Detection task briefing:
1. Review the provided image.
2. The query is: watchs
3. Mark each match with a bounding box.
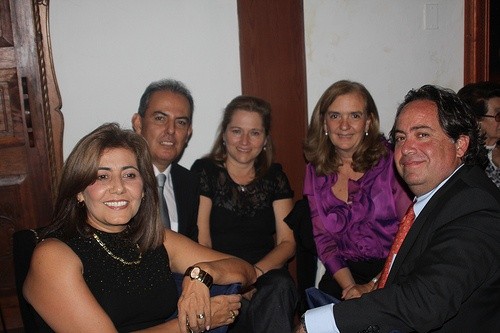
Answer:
[185,266,213,289]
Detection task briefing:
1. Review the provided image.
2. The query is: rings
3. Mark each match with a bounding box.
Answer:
[230,310,236,319]
[197,314,205,319]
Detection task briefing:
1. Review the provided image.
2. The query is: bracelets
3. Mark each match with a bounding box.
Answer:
[254,263,264,275]
[299,314,308,333]
[372,277,379,283]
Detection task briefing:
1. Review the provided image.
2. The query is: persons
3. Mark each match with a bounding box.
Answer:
[457,81,500,190]
[132,79,201,243]
[294,85,500,333]
[303,80,417,301]
[190,96,297,333]
[23,122,257,333]
[226,163,255,192]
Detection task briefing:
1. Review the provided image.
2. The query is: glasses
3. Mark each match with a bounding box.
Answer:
[482,113,500,122]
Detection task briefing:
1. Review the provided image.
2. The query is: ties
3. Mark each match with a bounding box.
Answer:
[375,199,418,289]
[155,174,171,228]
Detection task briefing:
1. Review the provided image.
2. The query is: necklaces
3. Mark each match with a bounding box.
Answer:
[92,224,142,265]
